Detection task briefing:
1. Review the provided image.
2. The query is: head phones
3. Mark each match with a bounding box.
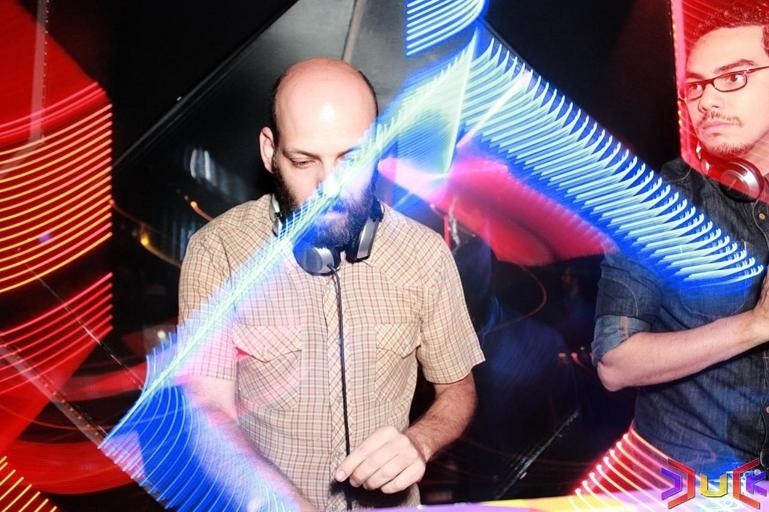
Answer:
[270,191,383,276]
[695,141,764,202]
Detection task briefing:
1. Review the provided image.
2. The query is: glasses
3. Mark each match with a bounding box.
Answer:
[682,66,769,100]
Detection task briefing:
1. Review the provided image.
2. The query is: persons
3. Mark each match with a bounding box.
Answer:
[170,52,489,512]
[579,0,769,485]
